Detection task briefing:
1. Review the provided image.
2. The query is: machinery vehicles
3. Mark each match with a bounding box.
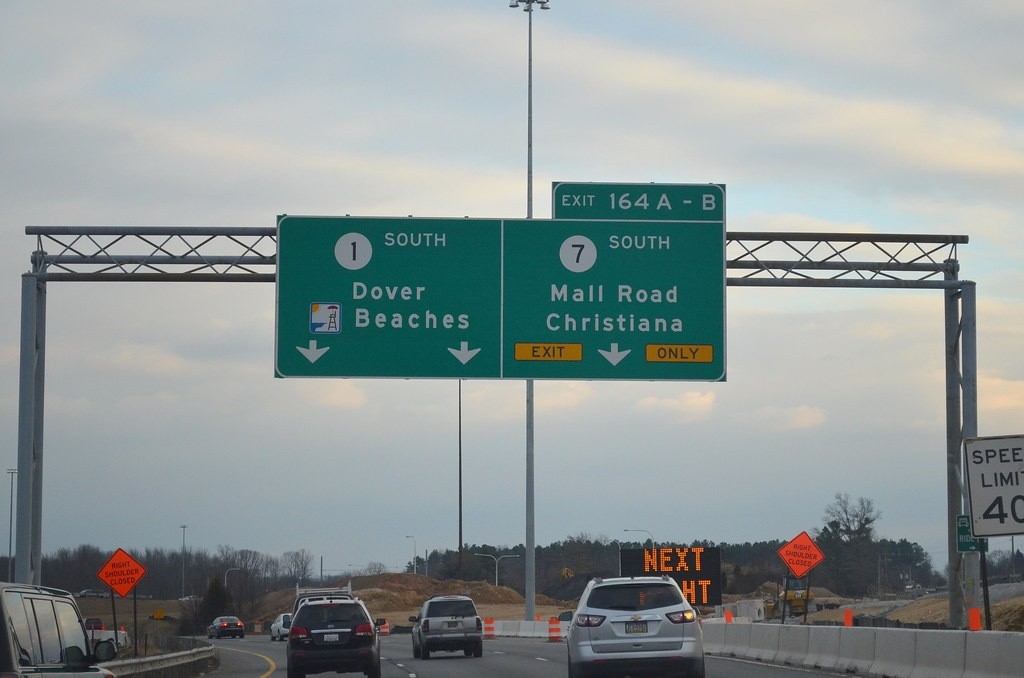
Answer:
[761,573,816,621]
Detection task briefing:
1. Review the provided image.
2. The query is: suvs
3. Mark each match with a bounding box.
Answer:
[408,593,484,660]
[558,572,705,678]
[282,590,386,678]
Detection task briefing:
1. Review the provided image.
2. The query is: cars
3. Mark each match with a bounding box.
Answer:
[74,587,154,602]
[207,616,245,640]
[270,612,294,641]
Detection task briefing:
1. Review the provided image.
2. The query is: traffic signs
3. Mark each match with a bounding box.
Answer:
[274,214,503,381]
[501,216,727,382]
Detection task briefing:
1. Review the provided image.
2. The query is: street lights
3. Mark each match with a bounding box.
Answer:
[877,557,892,597]
[224,567,241,588]
[623,529,655,549]
[6,469,19,581]
[180,524,188,598]
[406,534,417,574]
[347,564,367,577]
[474,553,520,587]
[605,539,622,577]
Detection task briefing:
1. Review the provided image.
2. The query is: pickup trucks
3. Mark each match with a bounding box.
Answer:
[0,581,132,678]
